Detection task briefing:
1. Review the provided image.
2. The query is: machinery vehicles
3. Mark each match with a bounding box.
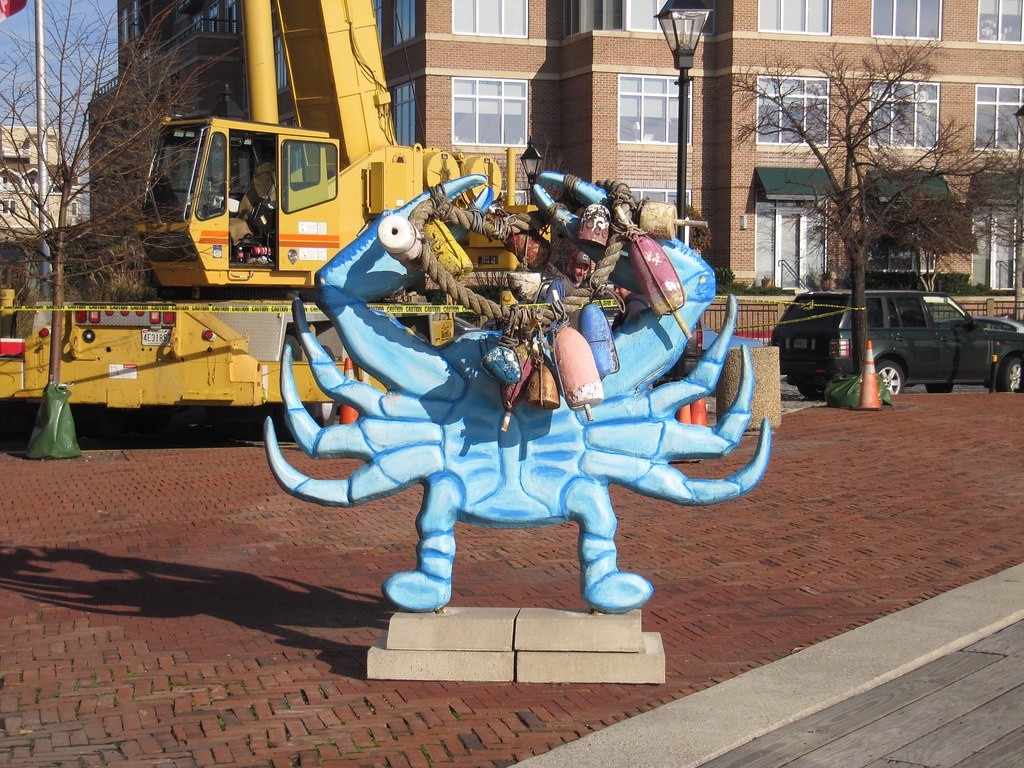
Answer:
[0,1,619,435]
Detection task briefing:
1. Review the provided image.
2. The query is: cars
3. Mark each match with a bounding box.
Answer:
[935,315,1023,332]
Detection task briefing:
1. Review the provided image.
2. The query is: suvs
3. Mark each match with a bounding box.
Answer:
[771,290,1024,394]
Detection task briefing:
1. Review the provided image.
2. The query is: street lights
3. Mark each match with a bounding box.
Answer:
[653,0,714,243]
[519,136,544,205]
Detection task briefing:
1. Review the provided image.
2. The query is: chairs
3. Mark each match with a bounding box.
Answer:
[228,158,277,247]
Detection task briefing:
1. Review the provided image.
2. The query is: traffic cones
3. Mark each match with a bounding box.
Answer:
[855,341,882,412]
